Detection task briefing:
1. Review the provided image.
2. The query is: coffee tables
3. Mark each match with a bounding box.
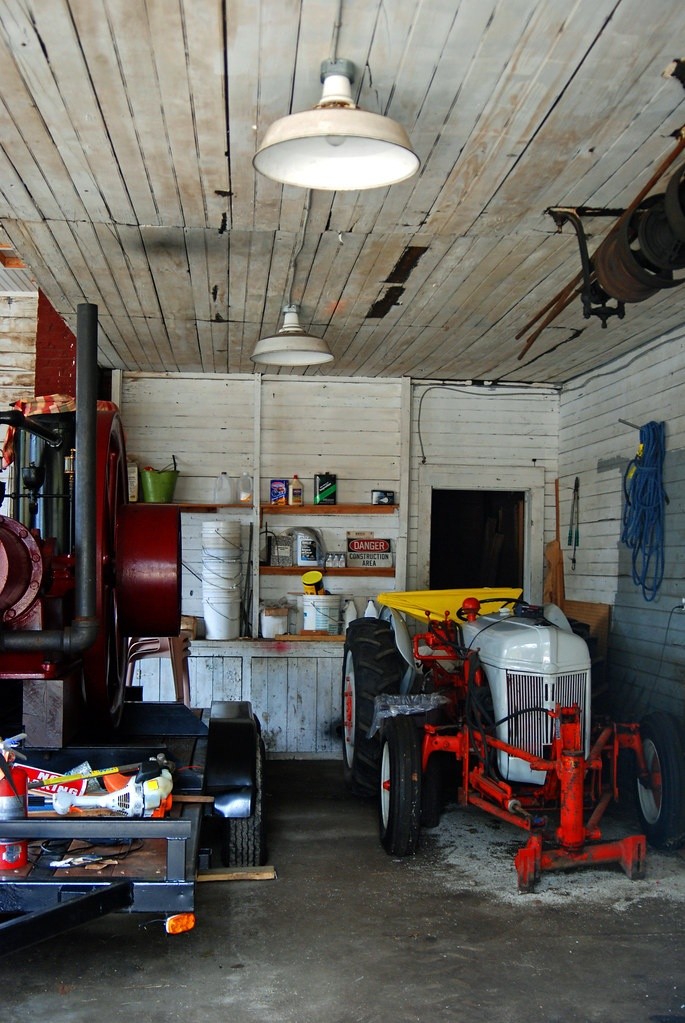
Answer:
[1,778,200,911]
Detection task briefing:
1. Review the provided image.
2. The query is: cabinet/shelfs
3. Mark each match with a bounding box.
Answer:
[255,499,398,641]
[118,498,253,641]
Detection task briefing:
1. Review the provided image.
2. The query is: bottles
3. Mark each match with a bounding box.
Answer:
[288,475,305,506]
[297,529,322,566]
[341,599,357,635]
[214,471,235,504]
[339,554,346,568]
[236,472,253,504]
[364,600,379,619]
[333,553,340,568]
[325,553,333,567]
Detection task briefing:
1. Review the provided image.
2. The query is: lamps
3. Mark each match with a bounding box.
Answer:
[250,304,334,367]
[253,57,421,191]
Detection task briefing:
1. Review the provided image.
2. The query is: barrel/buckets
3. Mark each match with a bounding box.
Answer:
[140,463,180,503]
[303,595,341,635]
[200,520,244,640]
